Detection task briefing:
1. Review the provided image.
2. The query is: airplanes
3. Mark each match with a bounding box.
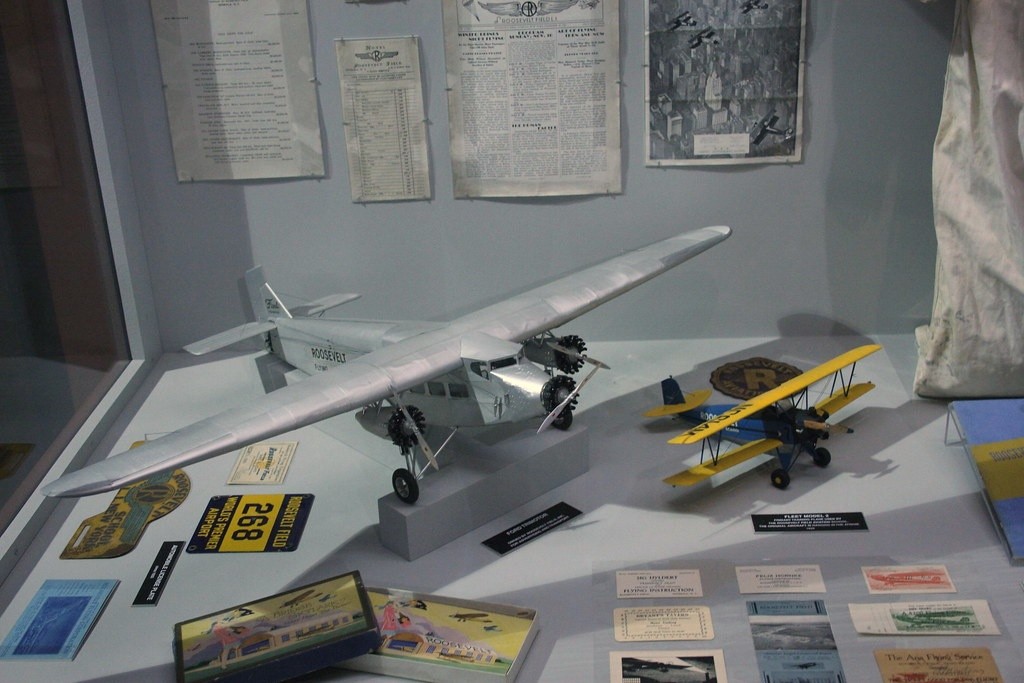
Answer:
[39,210,733,505]
[646,341,883,490]
